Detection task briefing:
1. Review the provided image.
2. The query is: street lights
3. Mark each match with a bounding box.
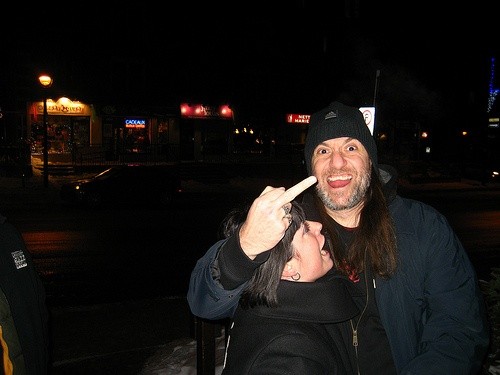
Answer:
[0,107,10,171]
[38,74,53,187]
[415,127,428,160]
[234,127,254,153]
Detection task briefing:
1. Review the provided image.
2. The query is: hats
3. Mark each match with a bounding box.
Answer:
[304,101,379,176]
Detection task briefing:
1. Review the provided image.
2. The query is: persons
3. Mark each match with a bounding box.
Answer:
[185,101,481,375]
[223,186,361,375]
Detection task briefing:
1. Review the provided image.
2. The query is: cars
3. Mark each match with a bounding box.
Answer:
[74,164,185,209]
